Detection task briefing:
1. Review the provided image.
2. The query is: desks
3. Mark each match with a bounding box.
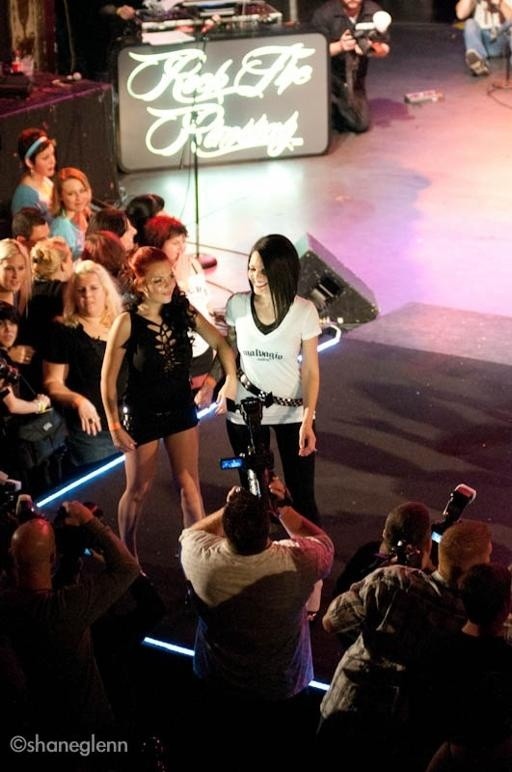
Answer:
[0,71,122,241]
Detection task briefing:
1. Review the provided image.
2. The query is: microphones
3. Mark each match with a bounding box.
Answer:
[491,17,511,45]
[49,72,82,86]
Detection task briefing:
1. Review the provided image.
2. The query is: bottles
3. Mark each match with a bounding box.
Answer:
[11,47,34,78]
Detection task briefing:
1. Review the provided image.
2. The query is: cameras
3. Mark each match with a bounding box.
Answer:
[199,14,222,40]
[55,498,101,547]
[430,483,476,562]
[348,19,388,58]
[0,356,20,385]
[219,391,274,498]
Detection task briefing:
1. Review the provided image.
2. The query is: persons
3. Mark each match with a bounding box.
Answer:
[1,0,512,769]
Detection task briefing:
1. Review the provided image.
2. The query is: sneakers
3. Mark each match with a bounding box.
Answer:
[465,49,489,75]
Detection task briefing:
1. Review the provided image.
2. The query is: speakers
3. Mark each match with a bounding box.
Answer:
[293,231,378,336]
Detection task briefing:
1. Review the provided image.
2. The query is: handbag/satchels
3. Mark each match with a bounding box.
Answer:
[20,411,69,463]
